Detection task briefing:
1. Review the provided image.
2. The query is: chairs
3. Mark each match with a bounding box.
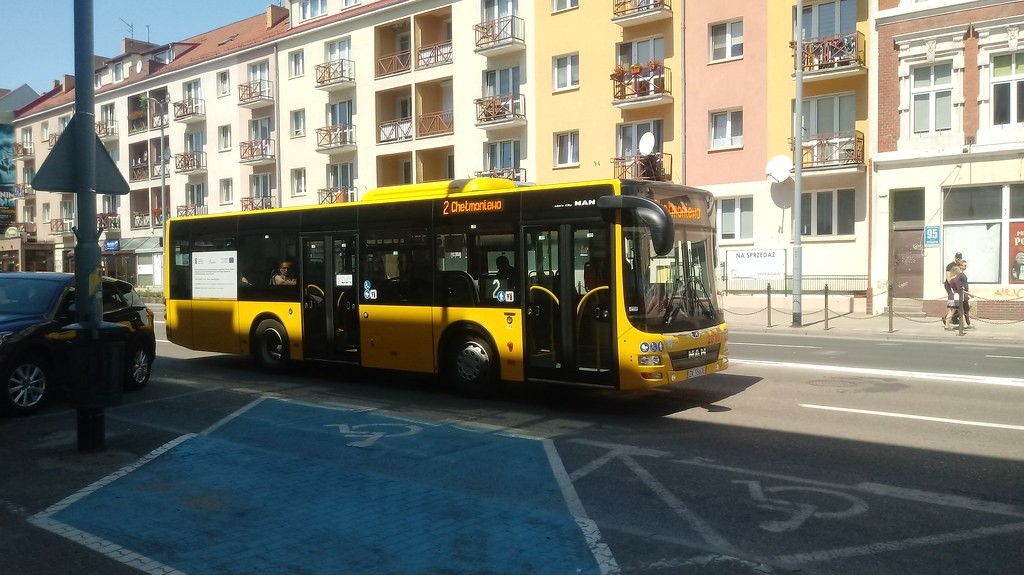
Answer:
[269,269,277,285]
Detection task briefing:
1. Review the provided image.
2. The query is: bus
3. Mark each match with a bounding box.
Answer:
[164,177,730,402]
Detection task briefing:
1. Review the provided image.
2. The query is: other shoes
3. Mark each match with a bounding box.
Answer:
[942,314,974,330]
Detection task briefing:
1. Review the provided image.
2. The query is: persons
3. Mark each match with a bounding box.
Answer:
[496,256,516,290]
[942,252,976,330]
[241,259,297,286]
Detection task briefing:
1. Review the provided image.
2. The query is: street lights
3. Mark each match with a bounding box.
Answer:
[141,96,167,250]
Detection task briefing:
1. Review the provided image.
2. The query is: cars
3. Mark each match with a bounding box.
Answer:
[0,272,157,418]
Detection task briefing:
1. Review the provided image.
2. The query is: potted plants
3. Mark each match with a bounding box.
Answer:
[647,59,658,71]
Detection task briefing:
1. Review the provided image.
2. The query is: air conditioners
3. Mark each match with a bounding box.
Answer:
[631,63,643,74]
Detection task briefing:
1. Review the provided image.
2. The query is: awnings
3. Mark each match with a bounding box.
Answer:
[69,237,163,256]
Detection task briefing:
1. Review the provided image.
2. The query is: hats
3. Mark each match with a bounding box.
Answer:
[955,252,962,258]
